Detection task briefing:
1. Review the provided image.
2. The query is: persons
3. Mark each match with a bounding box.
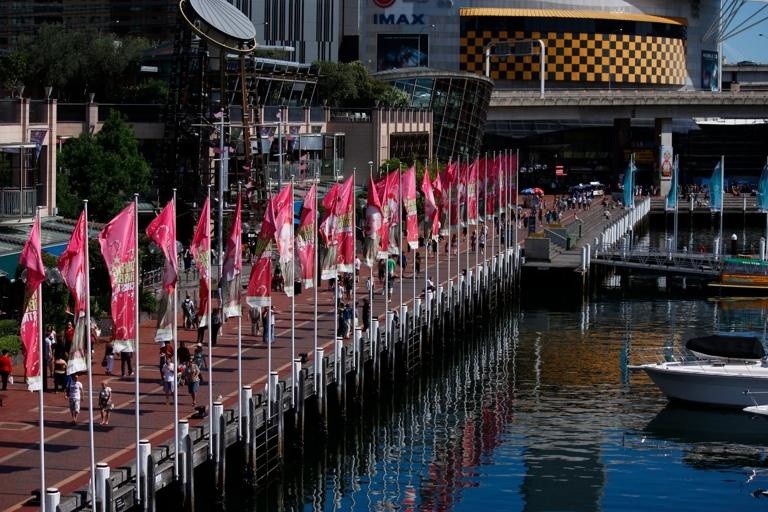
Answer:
[413,251,420,278]
[1,348,14,391]
[159,339,208,406]
[249,306,279,344]
[462,222,488,255]
[494,208,516,248]
[424,276,436,292]
[329,255,361,307]
[102,337,134,377]
[602,183,757,220]
[182,295,223,344]
[378,251,407,303]
[520,190,590,228]
[418,234,459,258]
[367,276,375,300]
[98,382,112,424]
[90,318,97,353]
[43,321,87,424]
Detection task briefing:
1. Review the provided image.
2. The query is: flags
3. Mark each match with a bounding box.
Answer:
[220,192,242,318]
[374,168,401,260]
[338,173,355,272]
[364,178,383,269]
[296,180,316,289]
[622,153,768,215]
[191,199,208,327]
[320,182,339,280]
[97,202,136,353]
[245,192,277,308]
[21,217,42,390]
[272,182,294,297]
[145,198,178,341]
[421,152,518,246]
[57,209,88,376]
[400,165,417,248]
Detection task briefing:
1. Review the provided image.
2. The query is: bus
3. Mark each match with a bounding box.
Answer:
[588,181,605,196]
[568,183,591,200]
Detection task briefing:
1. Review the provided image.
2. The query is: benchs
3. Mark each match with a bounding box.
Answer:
[560,199,622,239]
[694,192,760,208]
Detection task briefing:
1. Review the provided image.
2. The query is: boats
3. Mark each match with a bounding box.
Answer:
[623,401,767,471]
[706,257,768,289]
[740,391,767,418]
[625,331,767,407]
[706,296,767,329]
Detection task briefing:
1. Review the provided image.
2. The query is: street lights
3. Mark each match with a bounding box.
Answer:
[758,33,767,39]
[240,223,261,256]
[317,210,320,287]
[20,267,62,391]
[149,240,183,255]
[416,190,420,200]
[607,28,623,89]
[416,23,436,67]
